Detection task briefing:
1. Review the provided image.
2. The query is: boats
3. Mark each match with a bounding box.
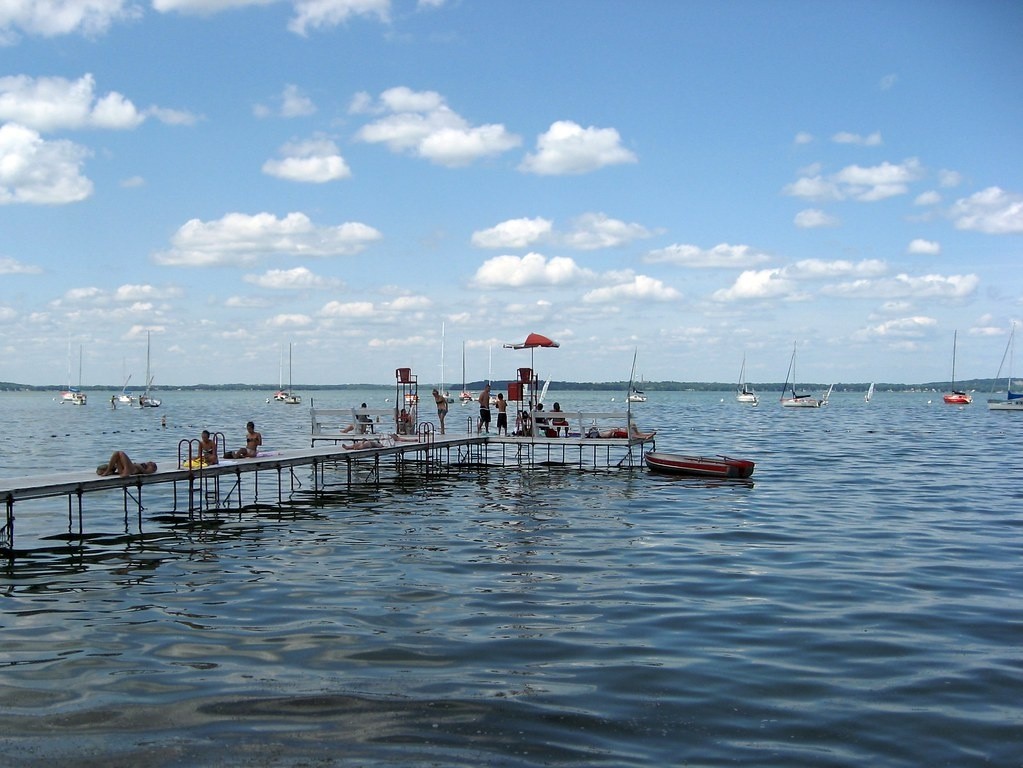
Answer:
[643,452,756,480]
[112,374,137,403]
[628,394,648,403]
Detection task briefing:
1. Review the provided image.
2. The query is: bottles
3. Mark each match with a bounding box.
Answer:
[376,415,379,422]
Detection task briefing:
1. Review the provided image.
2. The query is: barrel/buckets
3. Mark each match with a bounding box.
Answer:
[399,367,410,382]
[519,368,530,381]
[508,382,523,401]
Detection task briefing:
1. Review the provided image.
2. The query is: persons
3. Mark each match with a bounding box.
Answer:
[112,395,118,409]
[138,395,143,406]
[398,408,415,435]
[191,429,218,466]
[495,393,510,437]
[535,403,549,431]
[161,414,166,430]
[600,424,645,437]
[102,451,157,480]
[355,403,376,434]
[479,384,491,433]
[432,388,448,433]
[342,438,383,450]
[233,420,262,458]
[547,402,570,437]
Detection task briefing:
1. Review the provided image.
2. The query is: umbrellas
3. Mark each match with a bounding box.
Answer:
[503,333,561,411]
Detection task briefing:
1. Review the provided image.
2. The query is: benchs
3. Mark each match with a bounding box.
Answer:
[308,408,400,436]
[531,408,635,440]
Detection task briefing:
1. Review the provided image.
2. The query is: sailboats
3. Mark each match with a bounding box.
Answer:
[274,342,301,405]
[139,330,162,407]
[438,321,455,403]
[404,374,420,403]
[61,332,87,405]
[985,321,1023,410]
[459,341,543,406]
[780,341,823,407]
[943,330,973,403]
[735,359,757,402]
